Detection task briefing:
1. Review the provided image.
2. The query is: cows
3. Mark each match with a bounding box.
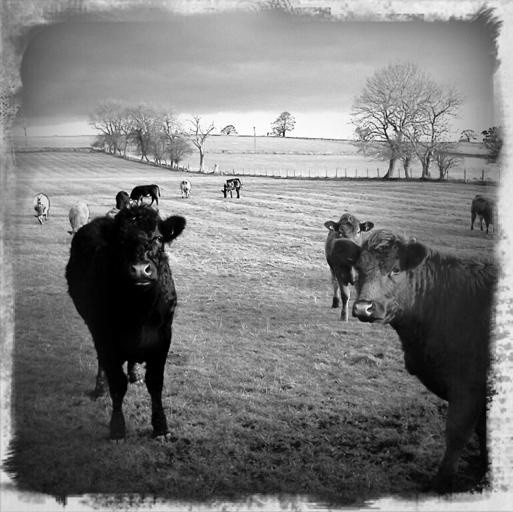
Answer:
[129,184,161,206]
[67,202,90,235]
[32,192,50,225]
[330,228,498,497]
[115,191,130,211]
[64,204,187,447]
[470,193,497,234]
[220,178,243,199]
[323,213,375,323]
[180,180,191,199]
